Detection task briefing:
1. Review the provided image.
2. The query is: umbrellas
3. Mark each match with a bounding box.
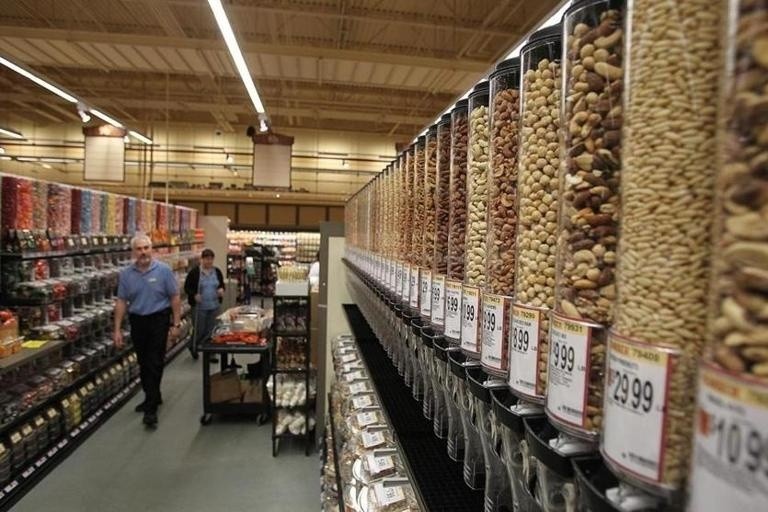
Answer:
[189,347,198,359]
[209,358,219,364]
[136,397,162,412]
[144,410,158,425]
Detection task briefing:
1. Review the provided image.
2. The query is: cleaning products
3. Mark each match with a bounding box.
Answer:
[196,318,272,427]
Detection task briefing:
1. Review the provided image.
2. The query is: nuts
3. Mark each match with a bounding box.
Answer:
[379,0,768,485]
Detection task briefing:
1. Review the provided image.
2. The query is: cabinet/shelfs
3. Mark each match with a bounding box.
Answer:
[221,229,321,283]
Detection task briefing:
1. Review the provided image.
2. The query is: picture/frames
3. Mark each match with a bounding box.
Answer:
[327,304,482,512]
[198,331,274,426]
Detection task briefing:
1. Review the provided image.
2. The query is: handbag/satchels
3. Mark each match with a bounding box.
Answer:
[174,323,181,328]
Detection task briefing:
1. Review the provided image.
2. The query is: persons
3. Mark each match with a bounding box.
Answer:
[183,248,227,363]
[111,233,183,428]
[309,247,320,297]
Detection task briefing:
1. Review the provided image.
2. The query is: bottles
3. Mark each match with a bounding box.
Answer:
[322,1,768,511]
[202,249,215,258]
[221,229,321,283]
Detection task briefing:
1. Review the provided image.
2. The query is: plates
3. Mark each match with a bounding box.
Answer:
[1,241,205,512]
[272,294,311,458]
